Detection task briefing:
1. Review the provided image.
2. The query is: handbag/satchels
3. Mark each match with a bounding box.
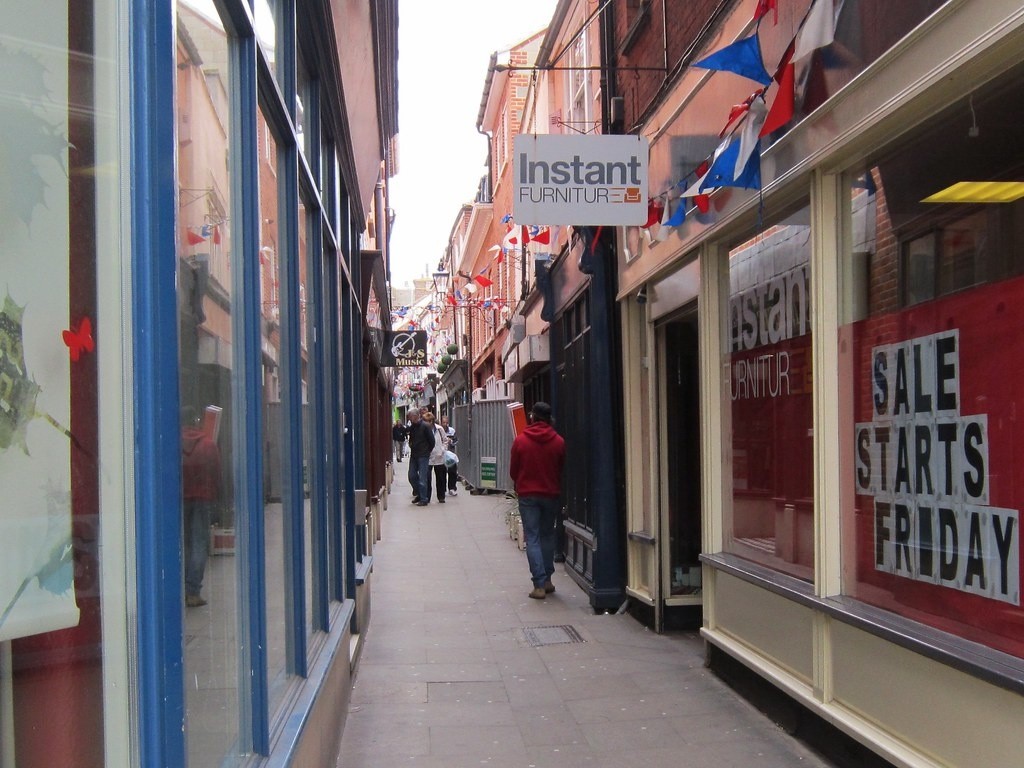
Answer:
[442,447,459,470]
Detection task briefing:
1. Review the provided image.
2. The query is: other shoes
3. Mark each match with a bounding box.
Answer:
[528,588,546,599]
[397,459,402,462]
[545,581,555,593]
[449,489,458,496]
[417,501,427,506]
[439,497,445,503]
[411,496,421,503]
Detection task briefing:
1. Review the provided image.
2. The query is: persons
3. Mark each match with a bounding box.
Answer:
[408,406,448,507]
[179,405,221,608]
[393,420,408,462]
[441,415,459,497]
[510,401,567,599]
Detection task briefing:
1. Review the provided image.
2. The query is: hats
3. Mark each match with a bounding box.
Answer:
[527,402,552,418]
